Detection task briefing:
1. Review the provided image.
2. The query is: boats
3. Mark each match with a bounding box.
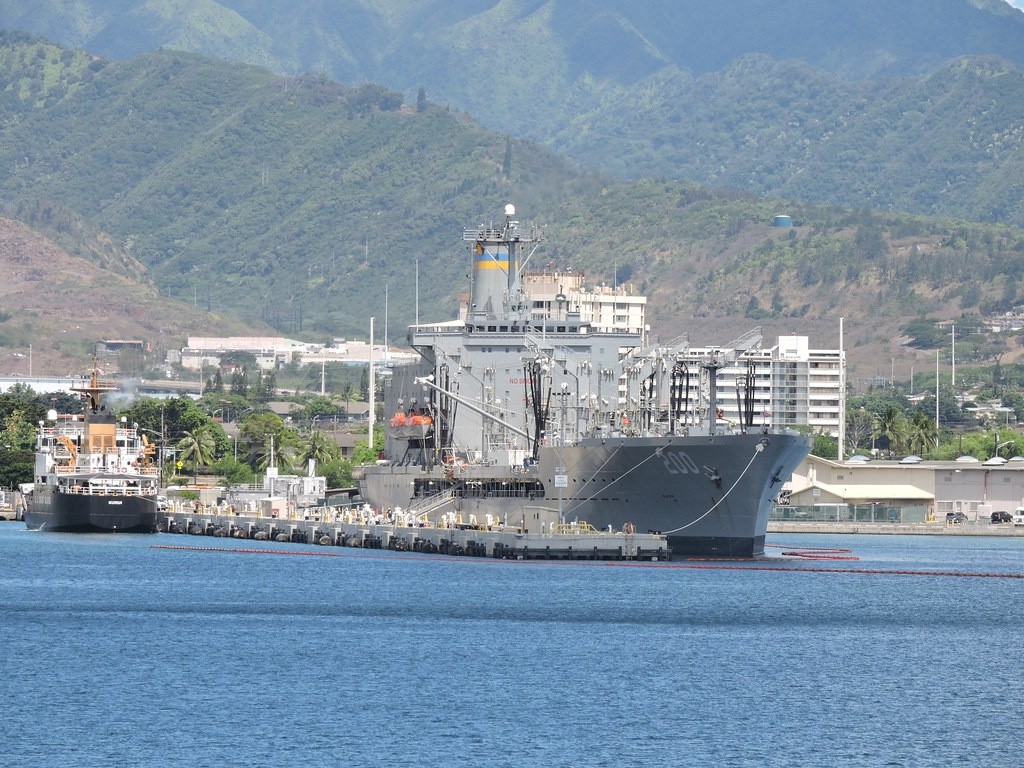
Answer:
[21,343,165,534]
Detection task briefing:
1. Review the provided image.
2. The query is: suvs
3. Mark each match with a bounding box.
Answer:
[991,511,1013,523]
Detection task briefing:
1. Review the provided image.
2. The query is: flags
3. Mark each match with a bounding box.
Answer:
[477,242,485,256]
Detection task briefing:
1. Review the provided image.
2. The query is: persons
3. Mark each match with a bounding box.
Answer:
[303,506,309,520]
[387,508,392,519]
[194,501,200,513]
[314,509,320,521]
[272,510,278,518]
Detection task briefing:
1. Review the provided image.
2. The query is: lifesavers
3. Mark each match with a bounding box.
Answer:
[121,467,127,473]
[272,510,278,517]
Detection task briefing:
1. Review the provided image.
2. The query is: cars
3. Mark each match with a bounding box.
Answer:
[945,512,968,523]
[1013,506,1024,527]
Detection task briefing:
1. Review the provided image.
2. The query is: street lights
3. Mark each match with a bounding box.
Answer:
[995,440,1015,457]
[205,403,340,467]
[141,427,164,489]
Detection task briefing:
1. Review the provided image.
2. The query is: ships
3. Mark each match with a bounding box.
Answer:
[357,199,816,559]
